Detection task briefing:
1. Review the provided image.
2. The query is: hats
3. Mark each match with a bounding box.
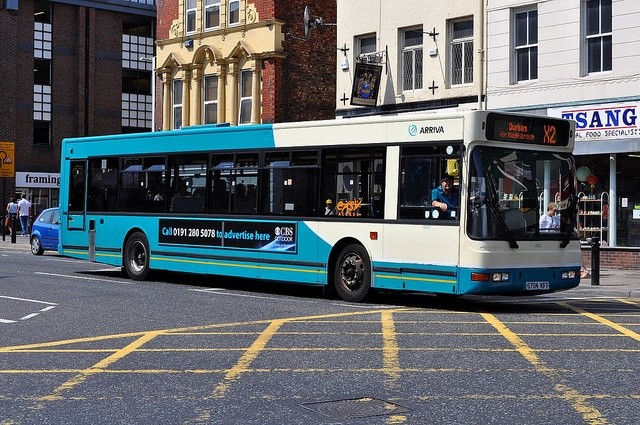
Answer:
[441,173,454,183]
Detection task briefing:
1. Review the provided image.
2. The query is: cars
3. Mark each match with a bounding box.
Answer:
[30,207,59,254]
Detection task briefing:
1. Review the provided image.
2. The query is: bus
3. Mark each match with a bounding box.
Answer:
[58,110,584,301]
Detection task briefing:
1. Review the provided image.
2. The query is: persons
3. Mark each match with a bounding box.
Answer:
[144,174,192,212]
[431,174,460,211]
[214,179,234,209]
[4,195,19,237]
[324,198,336,216]
[16,193,32,237]
[235,183,250,201]
[539,202,558,232]
